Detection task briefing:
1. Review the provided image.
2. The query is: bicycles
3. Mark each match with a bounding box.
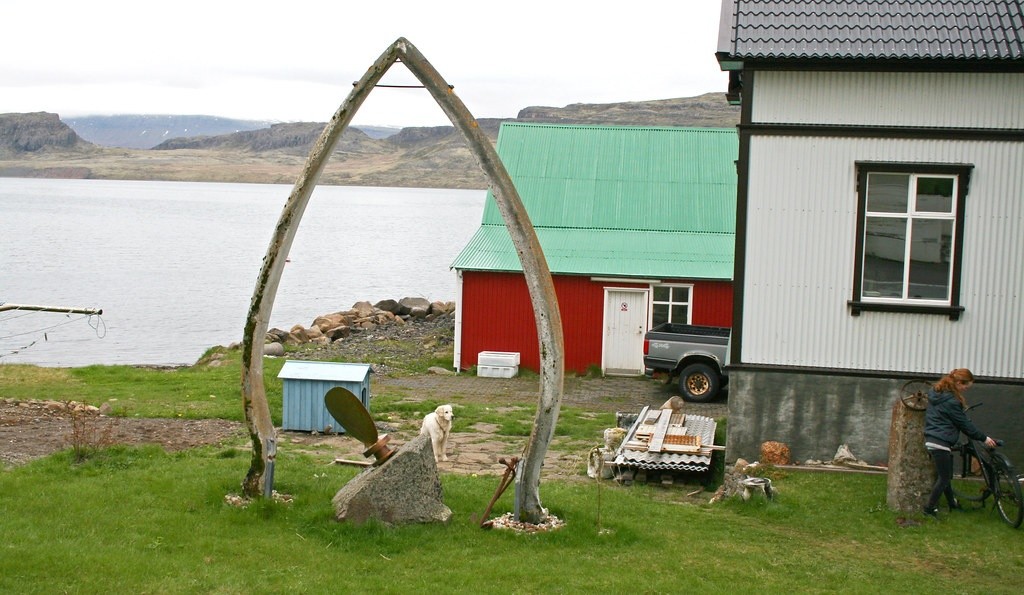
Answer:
[946,402,1024,532]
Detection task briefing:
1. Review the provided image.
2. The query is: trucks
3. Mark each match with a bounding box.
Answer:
[643,321,730,402]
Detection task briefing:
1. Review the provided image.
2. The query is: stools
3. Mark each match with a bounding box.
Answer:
[740,477,773,505]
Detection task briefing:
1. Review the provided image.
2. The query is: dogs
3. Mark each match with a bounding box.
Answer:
[420,404,454,463]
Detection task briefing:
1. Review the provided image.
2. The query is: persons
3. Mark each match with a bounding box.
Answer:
[922,369,996,522]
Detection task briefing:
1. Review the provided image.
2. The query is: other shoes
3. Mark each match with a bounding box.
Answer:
[920,506,941,519]
[949,499,965,512]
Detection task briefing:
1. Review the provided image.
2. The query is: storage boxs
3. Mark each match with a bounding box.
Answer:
[476,366,519,378]
[477,351,522,367]
